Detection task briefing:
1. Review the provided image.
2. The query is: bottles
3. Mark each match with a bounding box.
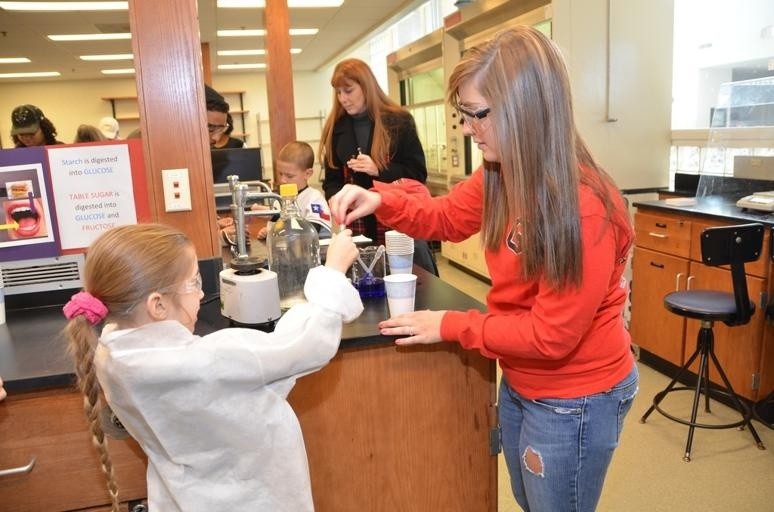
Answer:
[266,185,319,309]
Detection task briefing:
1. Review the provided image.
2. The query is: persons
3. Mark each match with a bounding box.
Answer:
[254,141,330,242]
[72,124,109,145]
[65,220,366,511]
[203,83,230,146]
[8,104,65,148]
[316,57,440,278]
[329,24,641,512]
[212,113,251,149]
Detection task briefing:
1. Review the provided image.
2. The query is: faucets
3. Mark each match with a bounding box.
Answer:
[235,184,284,256]
[227,174,274,204]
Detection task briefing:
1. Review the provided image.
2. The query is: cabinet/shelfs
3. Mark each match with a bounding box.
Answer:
[0,325,500,511]
[101,87,247,151]
[630,196,774,421]
[256,107,327,173]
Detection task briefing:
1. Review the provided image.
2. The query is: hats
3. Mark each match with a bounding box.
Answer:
[11,104,43,134]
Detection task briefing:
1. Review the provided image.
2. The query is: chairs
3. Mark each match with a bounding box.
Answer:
[637,221,766,460]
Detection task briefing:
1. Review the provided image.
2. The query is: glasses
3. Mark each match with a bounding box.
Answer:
[209,123,230,134]
[457,105,492,135]
[124,274,202,314]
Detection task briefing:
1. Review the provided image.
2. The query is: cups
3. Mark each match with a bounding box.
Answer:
[350,245,386,297]
[384,228,415,274]
[381,272,418,317]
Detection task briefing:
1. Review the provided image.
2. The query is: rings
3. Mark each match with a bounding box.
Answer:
[410,326,415,336]
